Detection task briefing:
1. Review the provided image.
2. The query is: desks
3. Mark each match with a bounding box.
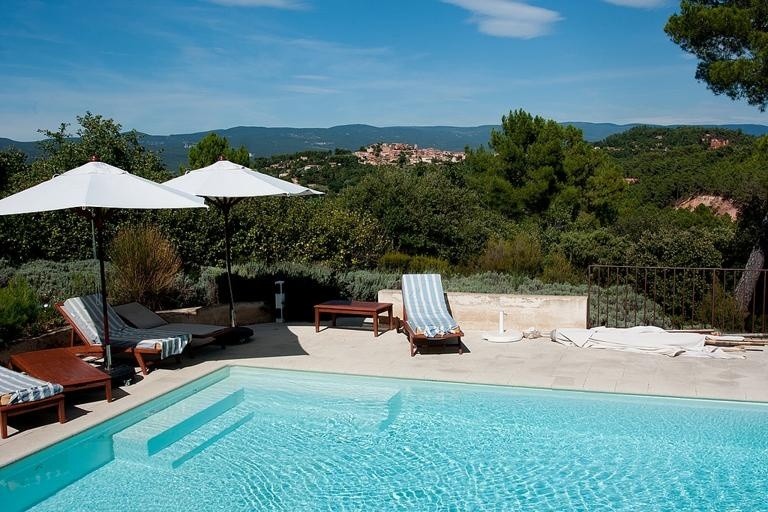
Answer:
[10,346,113,403]
[313,299,394,338]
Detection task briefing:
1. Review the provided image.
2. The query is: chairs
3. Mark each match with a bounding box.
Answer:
[398,272,464,356]
[0,363,68,440]
[54,291,255,376]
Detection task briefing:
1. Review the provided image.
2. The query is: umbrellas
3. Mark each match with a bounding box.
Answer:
[0,156,211,372]
[160,156,326,327]
